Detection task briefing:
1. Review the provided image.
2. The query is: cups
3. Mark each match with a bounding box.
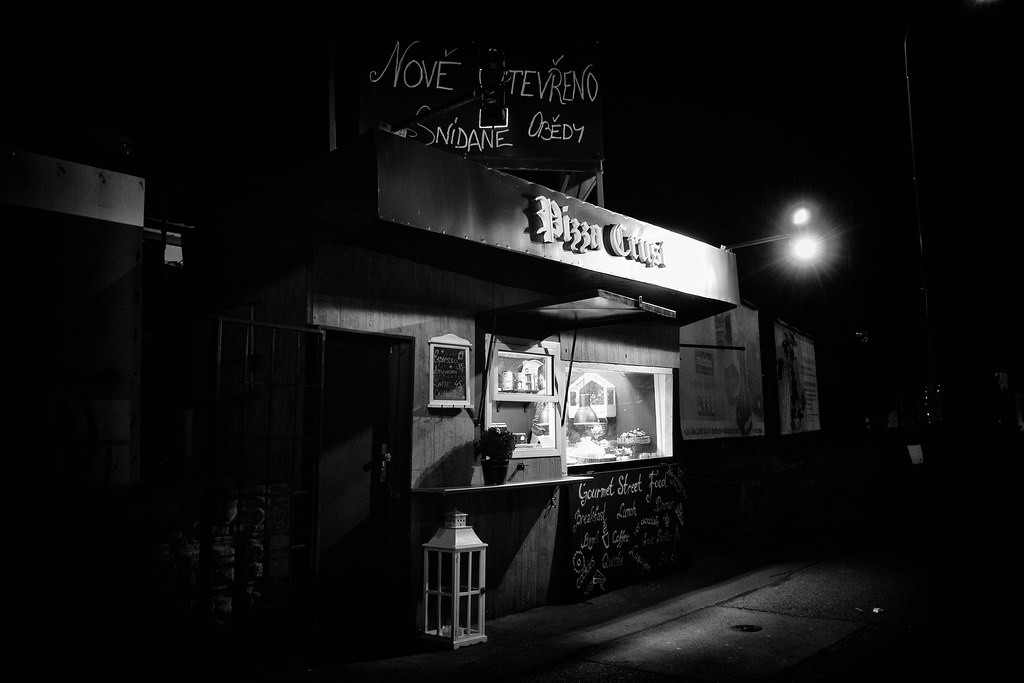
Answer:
[498,370,514,391]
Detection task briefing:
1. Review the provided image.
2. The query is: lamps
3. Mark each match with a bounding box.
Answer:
[573,373,598,424]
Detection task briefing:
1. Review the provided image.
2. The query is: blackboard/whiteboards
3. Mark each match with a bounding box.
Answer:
[365,1,607,173]
[564,463,686,605]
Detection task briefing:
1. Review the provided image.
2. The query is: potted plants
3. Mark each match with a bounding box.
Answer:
[474,428,517,485]
